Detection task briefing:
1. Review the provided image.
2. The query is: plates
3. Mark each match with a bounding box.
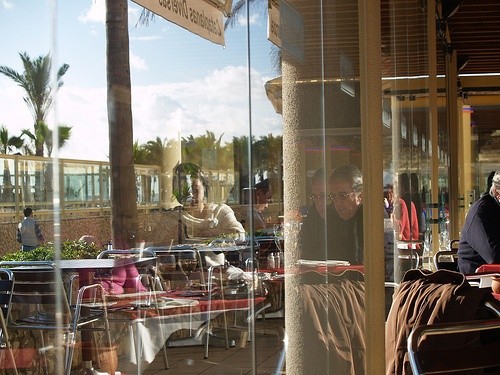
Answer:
[129,299,176,306]
[220,288,260,299]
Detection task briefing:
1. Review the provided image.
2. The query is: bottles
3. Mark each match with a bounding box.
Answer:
[269,252,275,269]
[274,252,280,269]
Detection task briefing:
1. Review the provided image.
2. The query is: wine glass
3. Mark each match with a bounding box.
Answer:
[156,254,176,299]
[179,251,198,301]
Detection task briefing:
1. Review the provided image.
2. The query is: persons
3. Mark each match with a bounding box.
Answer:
[457,171,500,277]
[439,186,448,221]
[154,162,275,325]
[302,166,422,278]
[16,208,46,252]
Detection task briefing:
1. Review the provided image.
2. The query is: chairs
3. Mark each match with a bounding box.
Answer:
[432,240,460,272]
[384,269,500,375]
[0,237,284,375]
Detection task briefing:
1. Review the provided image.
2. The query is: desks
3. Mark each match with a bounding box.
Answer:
[147,246,251,348]
[10,257,158,375]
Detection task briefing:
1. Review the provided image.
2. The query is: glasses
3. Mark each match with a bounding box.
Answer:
[311,194,327,200]
[328,192,352,200]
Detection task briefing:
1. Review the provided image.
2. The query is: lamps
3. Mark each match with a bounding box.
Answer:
[456,56,468,71]
[442,0,462,19]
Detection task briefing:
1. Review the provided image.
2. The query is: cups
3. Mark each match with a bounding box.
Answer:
[490,275,500,294]
[273,224,283,254]
